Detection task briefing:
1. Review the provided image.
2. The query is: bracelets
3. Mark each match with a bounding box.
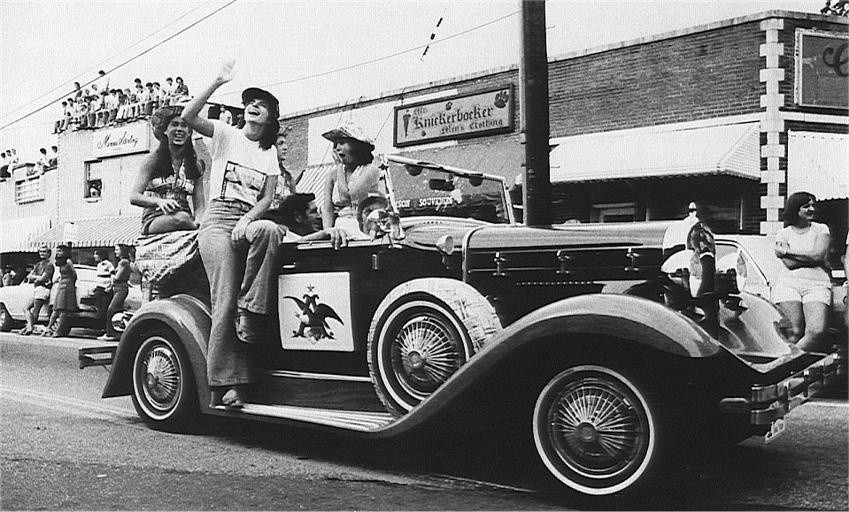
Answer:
[244,213,253,222]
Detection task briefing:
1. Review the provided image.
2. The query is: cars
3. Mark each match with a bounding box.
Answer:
[75,154,840,510]
[0,262,150,333]
[712,231,849,397]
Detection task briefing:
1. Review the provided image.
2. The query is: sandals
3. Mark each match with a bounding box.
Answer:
[222,387,243,408]
[233,308,256,344]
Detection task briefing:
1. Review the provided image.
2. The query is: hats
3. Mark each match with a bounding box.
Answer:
[242,88,279,106]
[151,105,185,141]
[358,191,388,212]
[321,122,376,151]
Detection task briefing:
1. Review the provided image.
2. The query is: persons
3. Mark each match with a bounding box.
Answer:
[0,244,131,341]
[403,109,411,136]
[774,192,831,352]
[281,193,348,251]
[129,106,206,234]
[322,125,390,242]
[0,71,245,183]
[259,126,296,226]
[181,58,285,409]
